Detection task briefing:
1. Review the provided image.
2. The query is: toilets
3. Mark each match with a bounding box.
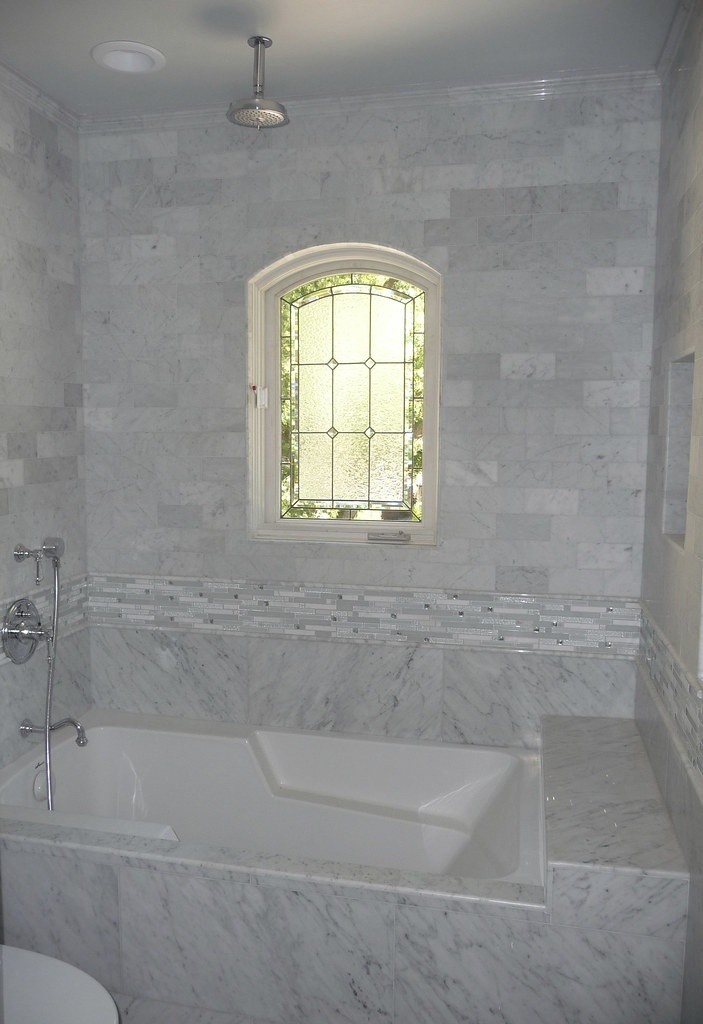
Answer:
[0,945,120,1024]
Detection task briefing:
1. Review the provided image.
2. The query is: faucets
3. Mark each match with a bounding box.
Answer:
[19,715,88,747]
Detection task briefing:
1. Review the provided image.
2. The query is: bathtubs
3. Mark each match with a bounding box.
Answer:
[0,709,544,1024]
